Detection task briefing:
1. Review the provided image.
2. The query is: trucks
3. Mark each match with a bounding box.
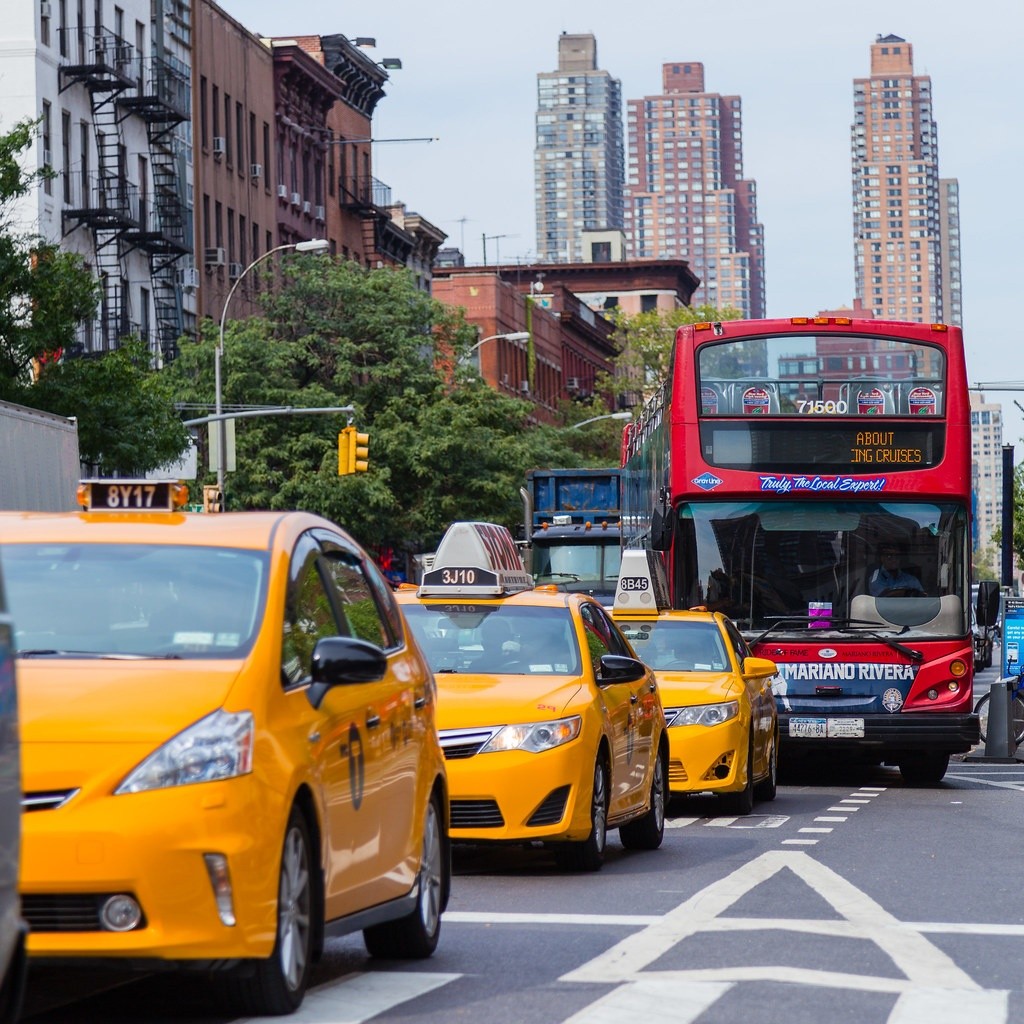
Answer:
[518,466,661,620]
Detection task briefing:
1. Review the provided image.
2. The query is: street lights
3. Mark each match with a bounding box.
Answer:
[448,332,531,393]
[217,238,331,499]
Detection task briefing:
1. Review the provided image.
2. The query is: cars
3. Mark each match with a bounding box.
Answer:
[971,583,1014,672]
[0,477,454,1016]
[590,549,780,819]
[378,520,673,874]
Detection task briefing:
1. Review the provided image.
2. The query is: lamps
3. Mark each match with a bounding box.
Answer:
[349,37,375,49]
[376,58,401,69]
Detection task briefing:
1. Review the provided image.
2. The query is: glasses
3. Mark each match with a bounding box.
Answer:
[883,553,901,560]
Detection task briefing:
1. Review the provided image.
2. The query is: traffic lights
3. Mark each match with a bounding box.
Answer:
[348,428,371,474]
[202,484,223,514]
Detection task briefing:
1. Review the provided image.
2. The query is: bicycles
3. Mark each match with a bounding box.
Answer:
[973,655,1024,745]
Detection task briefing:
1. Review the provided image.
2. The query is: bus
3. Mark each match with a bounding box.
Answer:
[618,313,1001,789]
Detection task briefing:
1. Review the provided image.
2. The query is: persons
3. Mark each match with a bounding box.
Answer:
[468,619,530,673]
[851,538,927,600]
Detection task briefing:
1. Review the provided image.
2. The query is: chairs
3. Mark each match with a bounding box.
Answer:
[840,377,944,416]
[626,376,782,465]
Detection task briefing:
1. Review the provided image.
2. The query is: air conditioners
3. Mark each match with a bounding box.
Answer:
[521,381,528,391]
[94,36,106,50]
[186,268,200,288]
[567,377,579,387]
[303,201,312,214]
[251,164,261,177]
[205,248,226,266]
[115,48,132,60]
[278,185,287,198]
[214,137,226,153]
[292,192,300,205]
[229,263,243,278]
[315,206,325,220]
